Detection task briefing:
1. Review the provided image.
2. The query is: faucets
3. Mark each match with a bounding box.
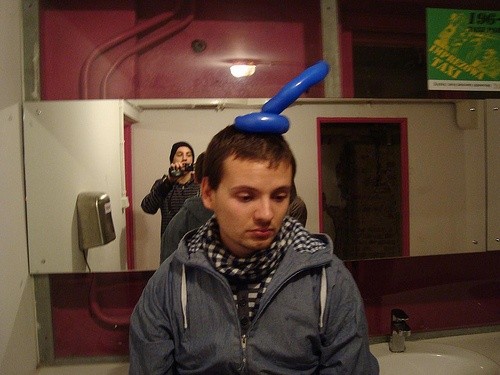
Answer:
[388,308,412,353]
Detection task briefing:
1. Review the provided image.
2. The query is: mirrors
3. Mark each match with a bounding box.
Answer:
[20,0,500,277]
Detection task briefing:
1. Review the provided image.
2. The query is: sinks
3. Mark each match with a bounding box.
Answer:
[368,342,499,375]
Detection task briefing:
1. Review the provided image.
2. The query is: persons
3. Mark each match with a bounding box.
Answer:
[129,123,380,375]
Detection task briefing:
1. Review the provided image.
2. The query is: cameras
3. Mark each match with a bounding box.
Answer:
[184,163,195,171]
